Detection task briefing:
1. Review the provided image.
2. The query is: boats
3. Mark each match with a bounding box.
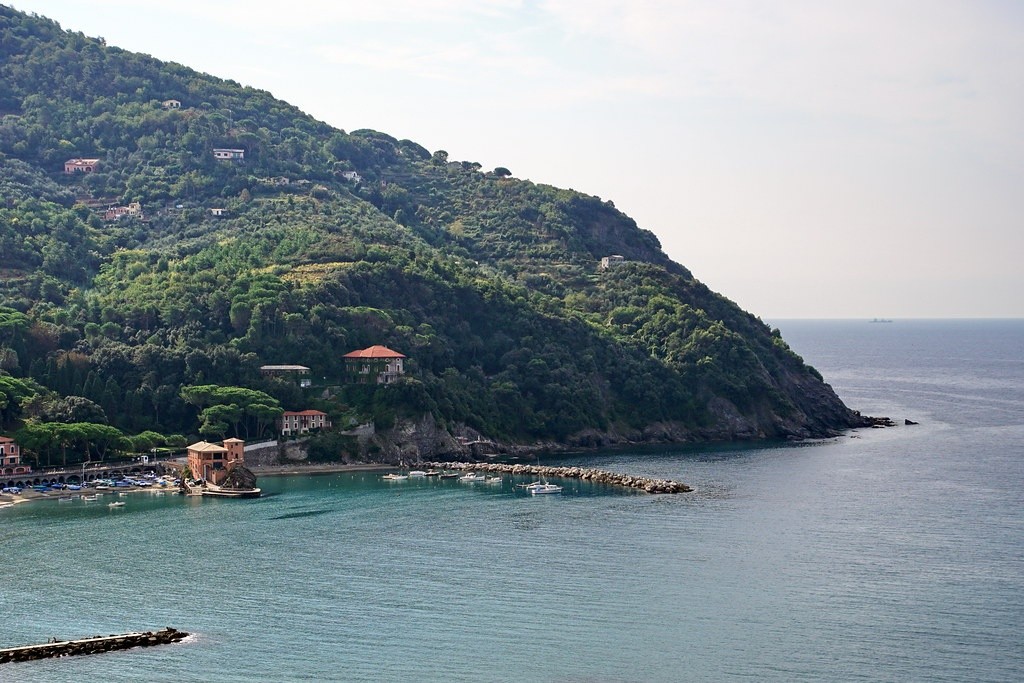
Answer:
[438,473,458,478]
[59,496,72,501]
[82,496,97,500]
[459,472,502,483]
[408,469,439,476]
[107,501,126,507]
[381,473,408,479]
[516,481,564,493]
